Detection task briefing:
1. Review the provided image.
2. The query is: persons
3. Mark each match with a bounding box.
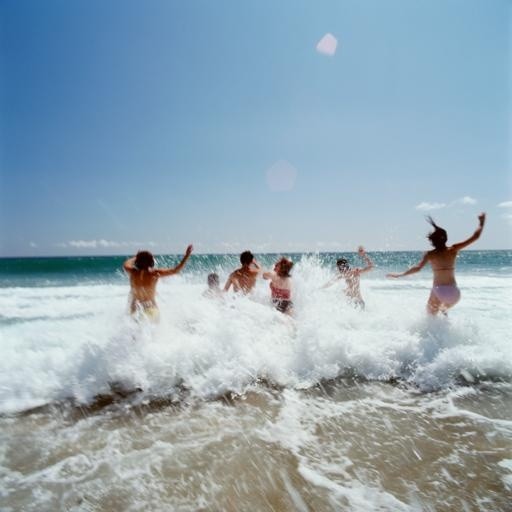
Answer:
[326,245,374,310]
[202,273,224,298]
[264,258,295,315]
[224,250,261,296]
[125,244,194,318]
[387,214,485,317]
